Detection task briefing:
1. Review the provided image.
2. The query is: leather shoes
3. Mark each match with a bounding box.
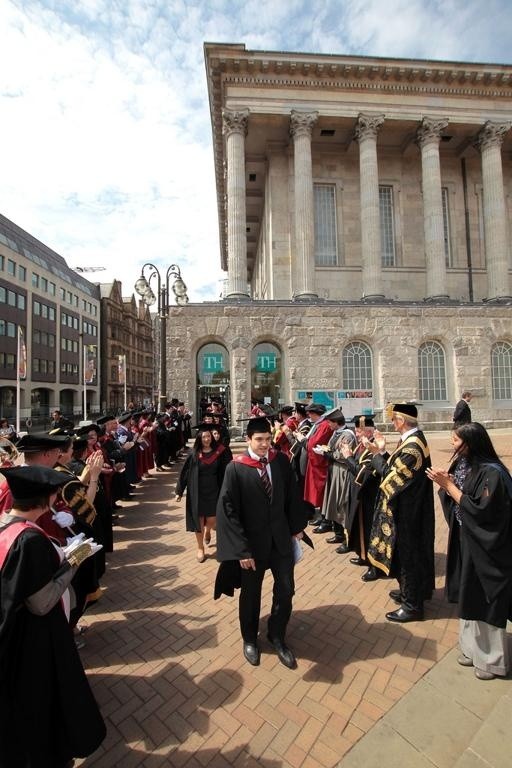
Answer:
[385,605,419,622]
[243,640,261,667]
[312,521,332,533]
[269,635,296,669]
[361,566,381,581]
[308,518,321,526]
[476,667,494,680]
[456,655,473,669]
[390,590,402,602]
[349,557,368,565]
[326,535,343,544]
[335,545,353,554]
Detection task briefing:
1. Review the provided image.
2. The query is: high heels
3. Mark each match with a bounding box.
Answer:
[205,535,210,545]
[195,549,207,562]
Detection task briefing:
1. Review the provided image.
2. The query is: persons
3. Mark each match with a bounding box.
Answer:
[83,424,115,553]
[51,410,73,432]
[129,401,194,486]
[98,415,124,512]
[118,416,136,502]
[107,415,132,501]
[196,401,232,536]
[311,408,360,554]
[450,391,474,428]
[293,403,332,534]
[64,428,107,578]
[0,418,19,457]
[216,418,304,671]
[52,432,104,635]
[177,424,227,565]
[362,402,435,621]
[0,433,94,656]
[245,403,311,463]
[339,414,385,581]
[427,422,511,681]
[0,466,111,768]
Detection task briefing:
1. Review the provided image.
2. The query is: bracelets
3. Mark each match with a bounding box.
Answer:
[89,480,99,483]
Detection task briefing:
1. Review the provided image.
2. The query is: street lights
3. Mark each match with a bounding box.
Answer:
[134,263,189,412]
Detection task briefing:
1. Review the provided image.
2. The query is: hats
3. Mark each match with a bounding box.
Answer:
[15,432,70,453]
[190,423,214,430]
[85,424,101,433]
[294,401,308,412]
[236,414,275,433]
[117,413,132,423]
[278,406,294,415]
[172,399,178,405]
[133,410,157,418]
[393,401,417,420]
[96,415,115,426]
[314,405,344,425]
[164,401,172,407]
[305,403,325,413]
[212,422,223,429]
[206,412,224,417]
[0,465,74,508]
[351,414,376,427]
[179,401,184,407]
